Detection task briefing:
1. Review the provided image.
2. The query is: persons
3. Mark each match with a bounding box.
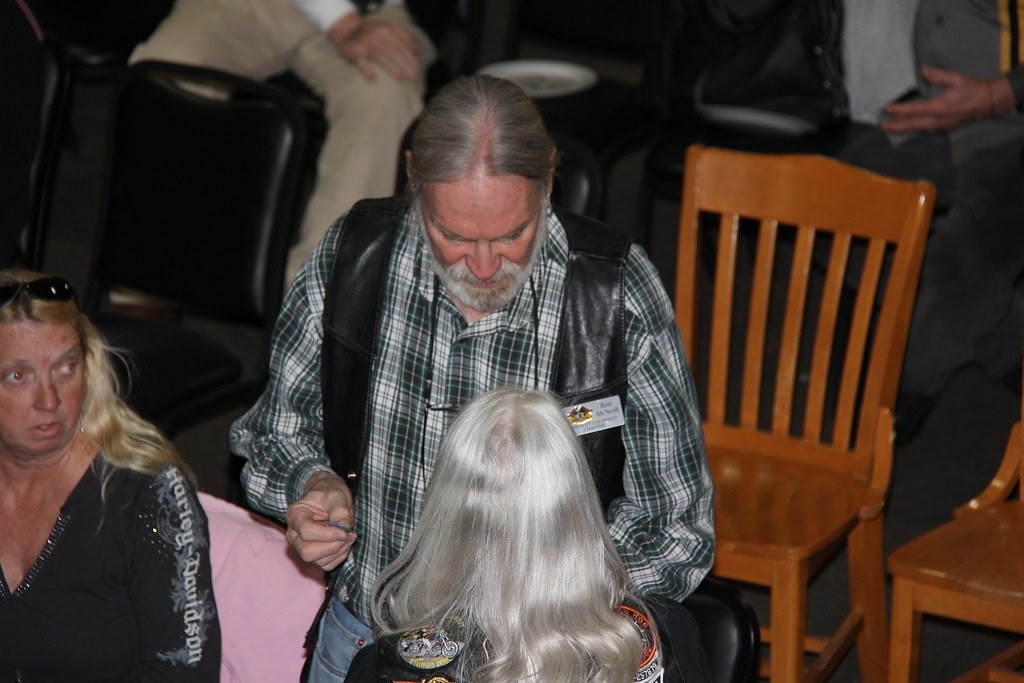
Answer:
[0,265,225,683]
[592,0,1024,610]
[230,72,717,683]
[124,0,438,300]
[340,387,716,683]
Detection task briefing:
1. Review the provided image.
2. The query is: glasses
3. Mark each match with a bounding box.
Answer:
[419,398,462,489]
[1,274,76,306]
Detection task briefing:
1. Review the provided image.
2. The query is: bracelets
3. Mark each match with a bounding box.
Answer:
[984,80,1000,119]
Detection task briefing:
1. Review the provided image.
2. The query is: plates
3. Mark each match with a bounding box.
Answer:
[476,58,599,100]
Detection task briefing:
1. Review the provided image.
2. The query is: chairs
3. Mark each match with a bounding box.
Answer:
[682,595,761,683]
[675,145,939,683]
[887,363,1024,683]
[78,58,328,443]
